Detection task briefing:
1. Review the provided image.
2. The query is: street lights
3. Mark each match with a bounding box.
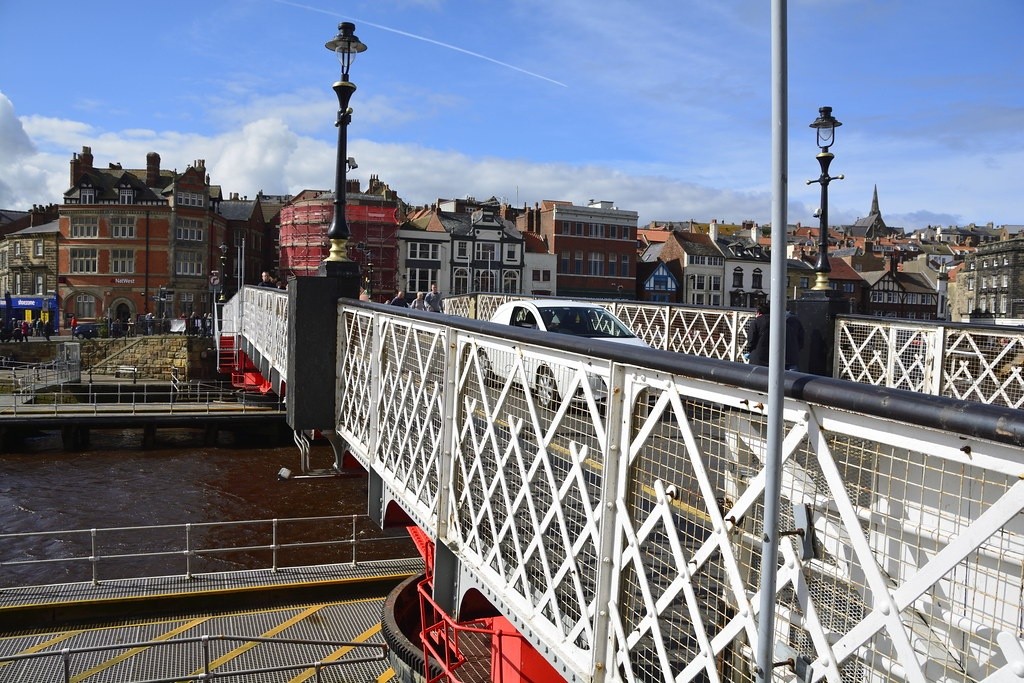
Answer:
[806,106,844,290]
[323,22,367,261]
[219,242,228,303]
[366,249,376,301]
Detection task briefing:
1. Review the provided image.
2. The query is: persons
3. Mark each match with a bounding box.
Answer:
[110,319,120,331]
[0,317,43,342]
[529,311,557,330]
[162,310,169,335]
[745,302,769,368]
[258,271,276,288]
[410,291,427,334]
[784,306,806,373]
[179,312,189,335]
[144,313,156,335]
[189,312,213,338]
[423,283,444,338]
[390,291,409,336]
[70,316,80,340]
[43,322,51,341]
[126,317,133,338]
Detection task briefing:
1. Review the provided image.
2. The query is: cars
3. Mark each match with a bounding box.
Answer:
[74,324,101,340]
[477,298,659,410]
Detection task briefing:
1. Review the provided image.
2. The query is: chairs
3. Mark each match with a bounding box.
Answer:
[518,310,538,328]
[540,313,558,332]
[560,310,579,334]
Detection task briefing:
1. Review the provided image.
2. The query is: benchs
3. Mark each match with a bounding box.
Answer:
[115,364,138,380]
